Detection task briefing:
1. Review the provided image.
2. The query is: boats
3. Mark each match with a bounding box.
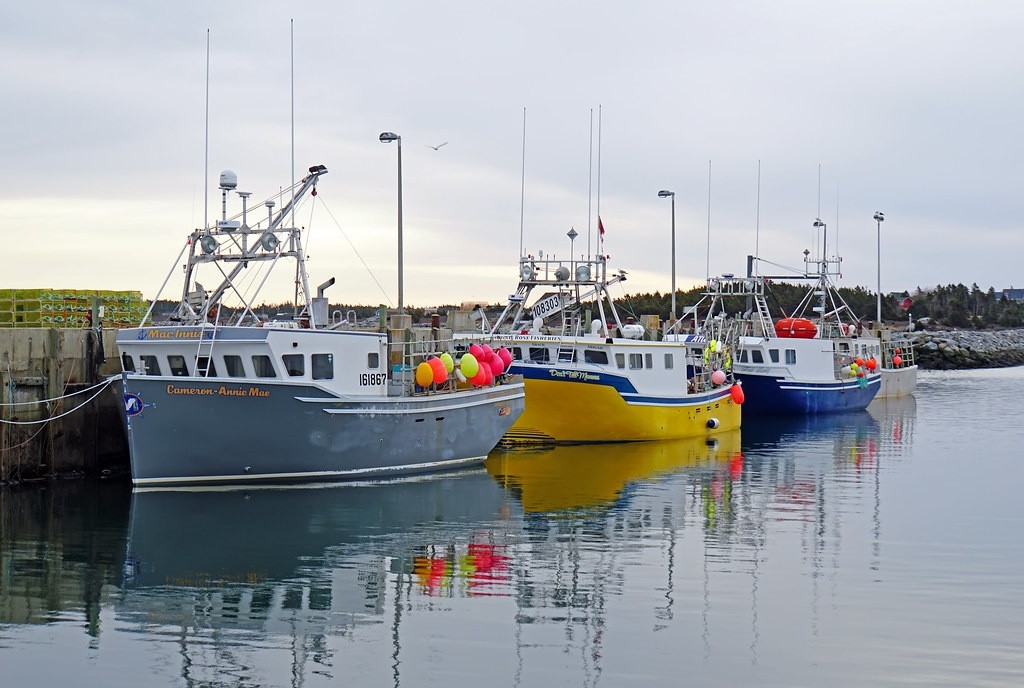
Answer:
[485,428,744,637]
[662,157,920,417]
[453,105,744,449]
[115,14,525,490]
[122,487,524,688]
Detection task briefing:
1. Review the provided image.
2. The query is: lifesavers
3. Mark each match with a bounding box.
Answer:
[840,325,849,334]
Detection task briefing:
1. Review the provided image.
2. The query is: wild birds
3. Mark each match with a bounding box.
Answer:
[426,142,448,150]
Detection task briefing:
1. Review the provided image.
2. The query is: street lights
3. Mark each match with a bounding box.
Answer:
[657,190,675,317]
[873,211,885,324]
[812,222,828,262]
[378,133,404,314]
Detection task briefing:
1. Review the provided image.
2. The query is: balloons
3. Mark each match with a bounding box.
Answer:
[841,348,903,378]
[416,344,513,387]
[712,370,746,406]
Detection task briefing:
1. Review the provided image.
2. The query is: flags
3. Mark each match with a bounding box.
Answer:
[598,215,605,235]
